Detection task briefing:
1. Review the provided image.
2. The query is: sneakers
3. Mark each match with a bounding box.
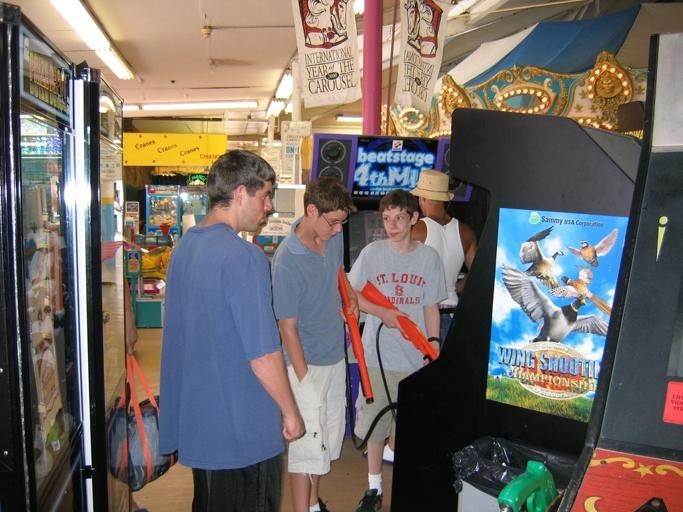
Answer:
[355,488,383,511]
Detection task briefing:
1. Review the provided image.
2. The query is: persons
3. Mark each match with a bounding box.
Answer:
[348,190,448,512]
[363,168,478,467]
[157,150,305,512]
[271,176,360,512]
[123,267,150,512]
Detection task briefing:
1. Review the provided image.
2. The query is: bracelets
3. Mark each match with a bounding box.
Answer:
[428,337,441,344]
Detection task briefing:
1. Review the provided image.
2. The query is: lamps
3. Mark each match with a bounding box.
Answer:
[121,99,257,113]
[50,0,134,82]
[335,113,363,122]
[262,69,292,119]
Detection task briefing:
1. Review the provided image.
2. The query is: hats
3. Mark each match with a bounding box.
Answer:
[410,169,455,202]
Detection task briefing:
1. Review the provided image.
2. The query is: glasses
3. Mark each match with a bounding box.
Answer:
[322,214,348,226]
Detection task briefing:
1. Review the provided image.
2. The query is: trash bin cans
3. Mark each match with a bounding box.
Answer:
[453,437,577,512]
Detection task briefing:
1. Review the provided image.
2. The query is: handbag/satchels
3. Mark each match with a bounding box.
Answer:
[105,396,128,483]
[127,394,178,491]
